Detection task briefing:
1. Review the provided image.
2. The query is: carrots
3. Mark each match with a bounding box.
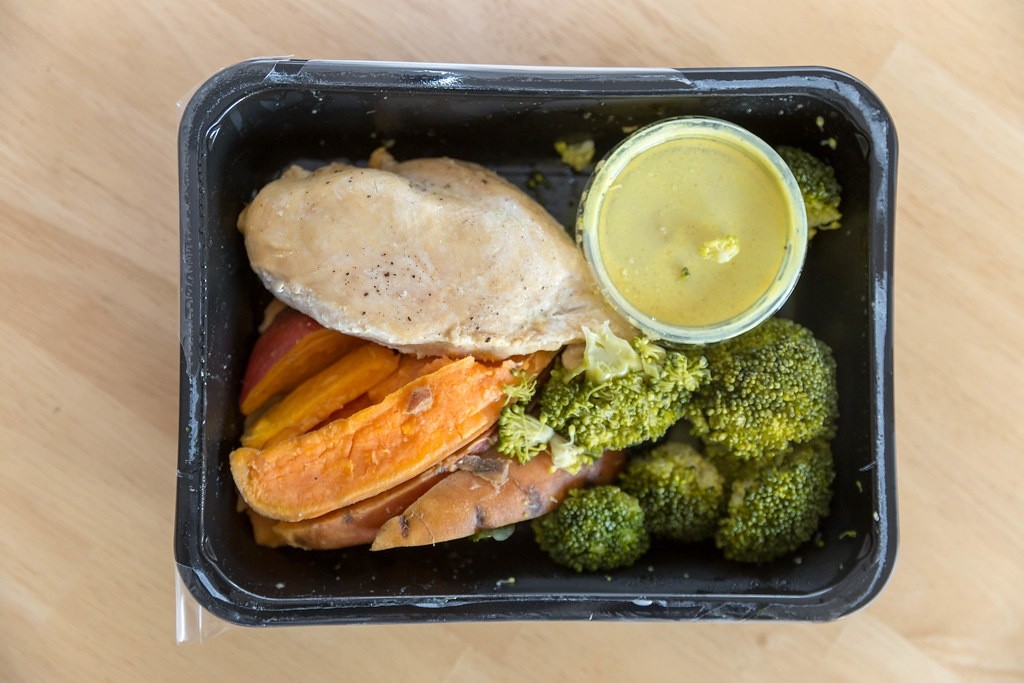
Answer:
[227,308,625,550]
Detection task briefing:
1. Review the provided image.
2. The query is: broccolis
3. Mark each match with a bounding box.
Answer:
[496,145,842,574]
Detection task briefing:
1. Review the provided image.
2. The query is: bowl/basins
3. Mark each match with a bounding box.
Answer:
[173,58,898,626]
[576,115,807,343]
